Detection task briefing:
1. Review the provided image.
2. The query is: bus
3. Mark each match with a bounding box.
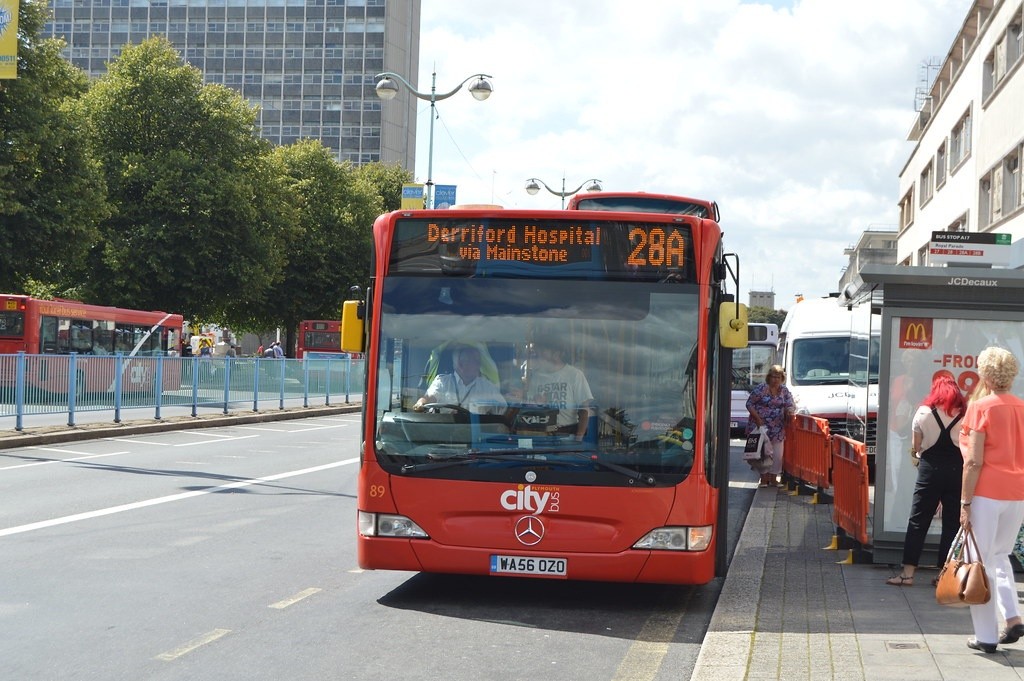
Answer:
[778,292,884,485]
[565,191,721,224]
[340,205,750,588]
[0,292,183,403]
[730,322,780,435]
[296,320,360,392]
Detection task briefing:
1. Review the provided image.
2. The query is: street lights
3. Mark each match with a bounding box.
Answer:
[375,59,494,211]
[524,166,603,210]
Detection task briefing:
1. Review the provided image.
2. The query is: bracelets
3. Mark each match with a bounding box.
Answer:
[960,500,970,507]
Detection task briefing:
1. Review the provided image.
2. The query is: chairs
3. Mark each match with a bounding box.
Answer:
[438,349,456,375]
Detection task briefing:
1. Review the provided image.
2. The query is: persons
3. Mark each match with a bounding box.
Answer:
[412,346,508,416]
[959,348,1024,652]
[227,345,237,382]
[522,338,595,442]
[197,337,214,384]
[257,341,284,382]
[182,338,193,378]
[745,364,797,487]
[886,375,969,586]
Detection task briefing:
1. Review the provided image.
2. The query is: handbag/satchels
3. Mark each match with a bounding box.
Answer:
[740,424,773,471]
[935,523,990,608]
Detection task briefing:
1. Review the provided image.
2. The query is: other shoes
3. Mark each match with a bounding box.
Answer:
[769,481,785,487]
[966,637,998,653]
[759,484,767,488]
[997,624,1024,645]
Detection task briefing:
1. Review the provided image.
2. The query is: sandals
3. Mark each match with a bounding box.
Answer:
[886,573,914,586]
[932,578,939,586]
